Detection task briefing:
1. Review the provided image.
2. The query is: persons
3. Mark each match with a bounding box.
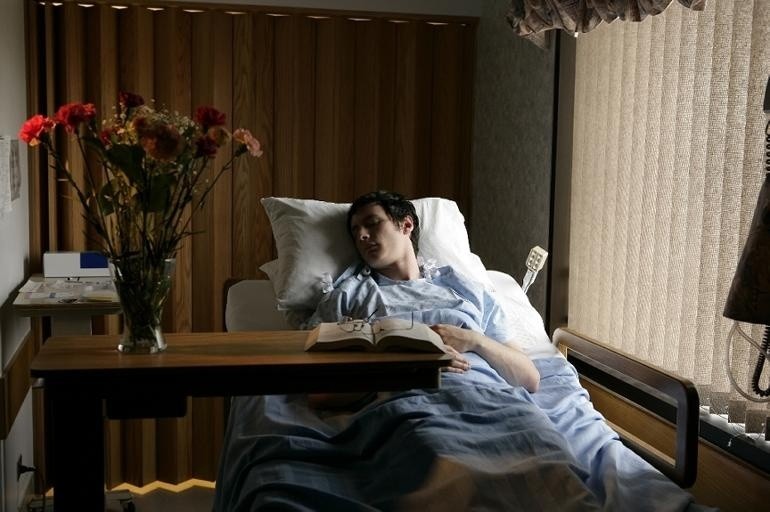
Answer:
[309,189,540,408]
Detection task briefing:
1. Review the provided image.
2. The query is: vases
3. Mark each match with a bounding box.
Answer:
[109,262,174,354]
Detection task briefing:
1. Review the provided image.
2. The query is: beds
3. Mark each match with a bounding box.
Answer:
[218,245,720,511]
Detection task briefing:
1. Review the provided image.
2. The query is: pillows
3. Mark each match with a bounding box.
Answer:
[259,195,493,312]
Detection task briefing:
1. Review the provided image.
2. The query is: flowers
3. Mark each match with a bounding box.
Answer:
[17,90,265,341]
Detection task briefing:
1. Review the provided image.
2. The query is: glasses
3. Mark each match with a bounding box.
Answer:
[337,309,413,335]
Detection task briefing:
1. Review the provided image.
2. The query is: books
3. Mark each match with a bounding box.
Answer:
[303,315,447,354]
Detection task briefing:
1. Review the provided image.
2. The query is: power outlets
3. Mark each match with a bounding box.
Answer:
[14,453,28,484]
